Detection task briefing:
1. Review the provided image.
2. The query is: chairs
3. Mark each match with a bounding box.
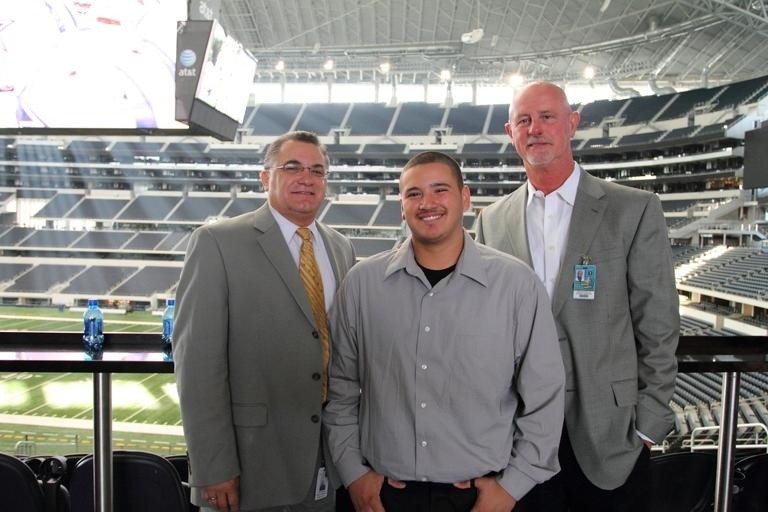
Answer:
[649,449,767,512]
[0,449,191,511]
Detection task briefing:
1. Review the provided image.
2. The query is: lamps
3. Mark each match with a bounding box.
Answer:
[383,68,457,112]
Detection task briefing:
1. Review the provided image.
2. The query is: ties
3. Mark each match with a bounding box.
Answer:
[296,228,330,404]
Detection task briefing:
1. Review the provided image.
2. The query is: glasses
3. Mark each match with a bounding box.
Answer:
[265,163,330,178]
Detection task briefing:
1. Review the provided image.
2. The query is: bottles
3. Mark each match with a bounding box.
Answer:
[82,298,104,358]
[161,299,176,360]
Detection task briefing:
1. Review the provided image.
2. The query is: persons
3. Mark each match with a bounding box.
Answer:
[170,129,357,511]
[575,270,585,281]
[472,80,680,511]
[322,150,567,512]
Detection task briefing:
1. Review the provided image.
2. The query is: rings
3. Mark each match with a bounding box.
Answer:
[208,497,216,502]
